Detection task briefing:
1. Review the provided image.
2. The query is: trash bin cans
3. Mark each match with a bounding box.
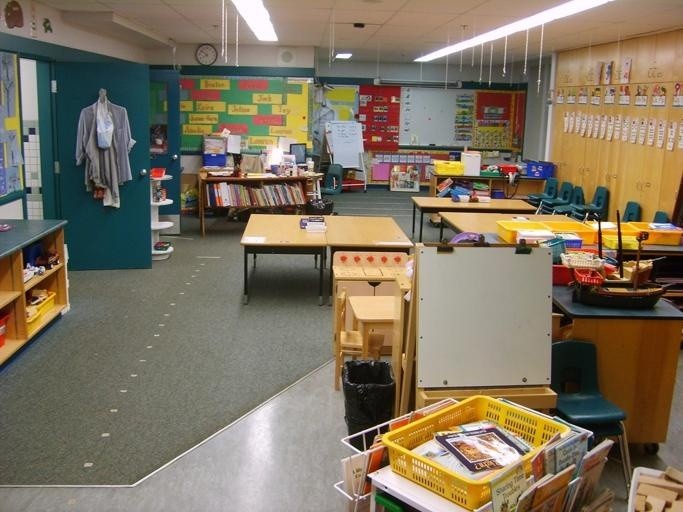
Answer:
[341,360,396,451]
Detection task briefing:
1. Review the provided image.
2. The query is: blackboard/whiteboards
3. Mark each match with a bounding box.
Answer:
[313,77,528,152]
[333,121,363,169]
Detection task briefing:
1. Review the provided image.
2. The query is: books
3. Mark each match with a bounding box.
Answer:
[299,216,326,233]
[342,407,614,511]
[204,181,306,206]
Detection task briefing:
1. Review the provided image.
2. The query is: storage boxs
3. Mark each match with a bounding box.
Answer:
[201,133,226,166]
[460,153,480,177]
[527,162,555,178]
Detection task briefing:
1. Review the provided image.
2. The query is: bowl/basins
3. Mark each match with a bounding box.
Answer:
[151,167,165,178]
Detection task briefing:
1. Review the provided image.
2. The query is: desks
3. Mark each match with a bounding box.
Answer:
[346,294,402,360]
[240,213,414,306]
[412,196,535,241]
[437,212,576,242]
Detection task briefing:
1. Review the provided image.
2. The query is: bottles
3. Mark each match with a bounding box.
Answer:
[153,188,165,201]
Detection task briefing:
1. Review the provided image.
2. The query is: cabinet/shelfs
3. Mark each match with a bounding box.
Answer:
[0,219,71,361]
[197,167,322,237]
[624,252,683,300]
[150,175,173,262]
[429,171,546,201]
[332,251,408,295]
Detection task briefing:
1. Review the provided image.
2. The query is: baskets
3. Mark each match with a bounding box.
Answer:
[480,164,517,176]
[542,232,603,286]
[381,394,572,510]
[434,160,464,176]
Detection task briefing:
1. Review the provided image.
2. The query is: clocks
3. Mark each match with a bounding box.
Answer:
[195,43,218,67]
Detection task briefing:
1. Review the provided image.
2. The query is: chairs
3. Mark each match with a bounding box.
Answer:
[551,339,637,501]
[330,294,385,390]
[527,176,669,222]
[322,164,342,194]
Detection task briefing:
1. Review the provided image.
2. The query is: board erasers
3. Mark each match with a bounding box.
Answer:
[429,144,436,146]
